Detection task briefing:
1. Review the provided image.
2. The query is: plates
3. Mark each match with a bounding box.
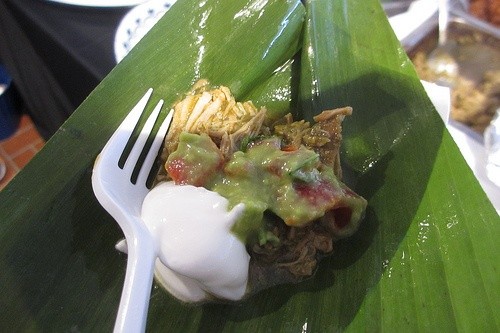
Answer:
[113,2,175,63]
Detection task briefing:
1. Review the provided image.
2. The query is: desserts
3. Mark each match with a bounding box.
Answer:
[140,80,368,304]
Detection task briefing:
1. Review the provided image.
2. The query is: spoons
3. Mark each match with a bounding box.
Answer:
[427,0,460,79]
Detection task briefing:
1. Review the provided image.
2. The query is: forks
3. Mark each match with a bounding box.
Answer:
[92,85,175,332]
